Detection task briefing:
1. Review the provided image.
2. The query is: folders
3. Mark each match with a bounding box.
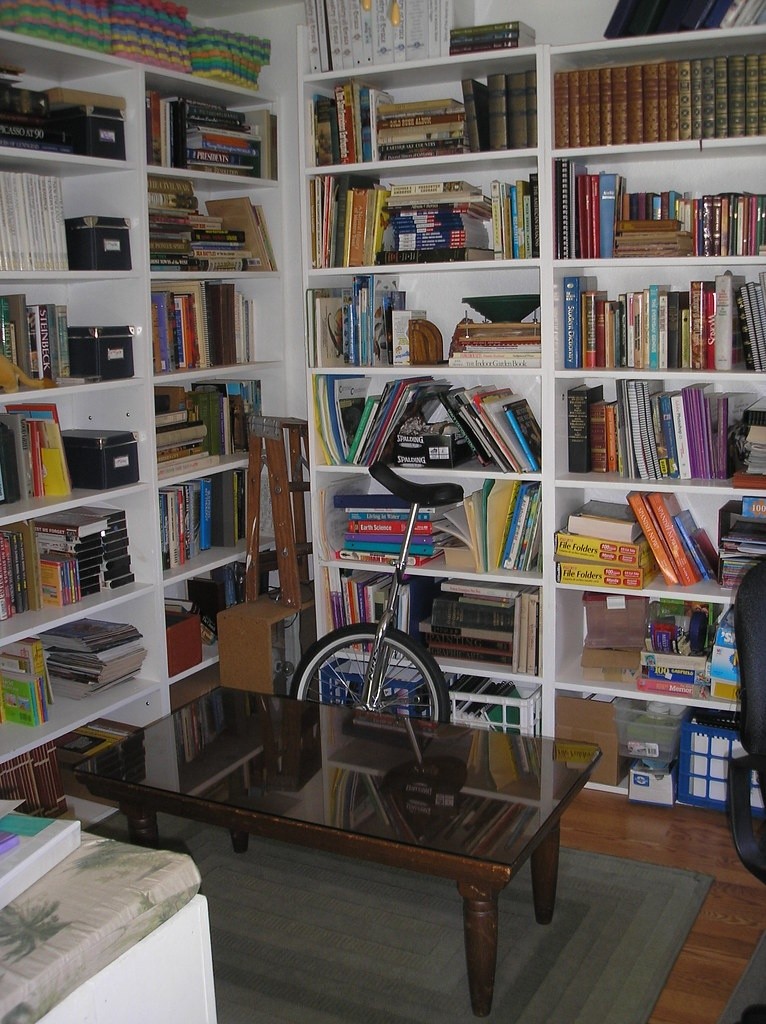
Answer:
[0,811,83,911]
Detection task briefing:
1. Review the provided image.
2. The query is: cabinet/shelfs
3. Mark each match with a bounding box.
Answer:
[0,807,219,1024]
[217,581,320,699]
[0,30,293,848]
[296,24,766,814]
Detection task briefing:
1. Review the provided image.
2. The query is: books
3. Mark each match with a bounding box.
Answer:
[0,618,148,727]
[309,173,541,270]
[563,271,766,373]
[311,373,542,475]
[554,53,766,151]
[0,170,69,272]
[567,379,766,490]
[553,491,766,590]
[76,685,542,859]
[164,560,246,678]
[604,0,766,40]
[554,158,766,260]
[52,717,147,787]
[0,500,135,621]
[0,62,125,155]
[0,402,72,506]
[322,565,543,735]
[0,740,68,818]
[308,69,538,167]
[316,475,543,574]
[306,273,542,369]
[304,0,536,74]
[146,89,278,571]
[0,294,102,384]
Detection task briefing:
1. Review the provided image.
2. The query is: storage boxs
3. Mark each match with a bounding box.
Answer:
[63,216,134,271]
[555,522,660,590]
[167,611,204,677]
[553,591,766,818]
[68,325,135,384]
[323,660,539,737]
[0,83,127,160]
[60,428,141,490]
[717,500,766,591]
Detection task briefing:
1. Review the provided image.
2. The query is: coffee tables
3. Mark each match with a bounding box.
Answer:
[71,686,604,1019]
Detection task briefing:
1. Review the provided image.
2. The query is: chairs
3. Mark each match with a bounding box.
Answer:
[725,559,766,1024]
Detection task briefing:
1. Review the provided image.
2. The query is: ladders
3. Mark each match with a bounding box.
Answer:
[244,415,314,608]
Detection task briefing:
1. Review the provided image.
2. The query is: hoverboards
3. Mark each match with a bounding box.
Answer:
[290,463,464,719]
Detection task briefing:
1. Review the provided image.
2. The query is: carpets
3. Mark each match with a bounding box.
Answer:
[717,931,765,1023]
[115,796,714,1024]
